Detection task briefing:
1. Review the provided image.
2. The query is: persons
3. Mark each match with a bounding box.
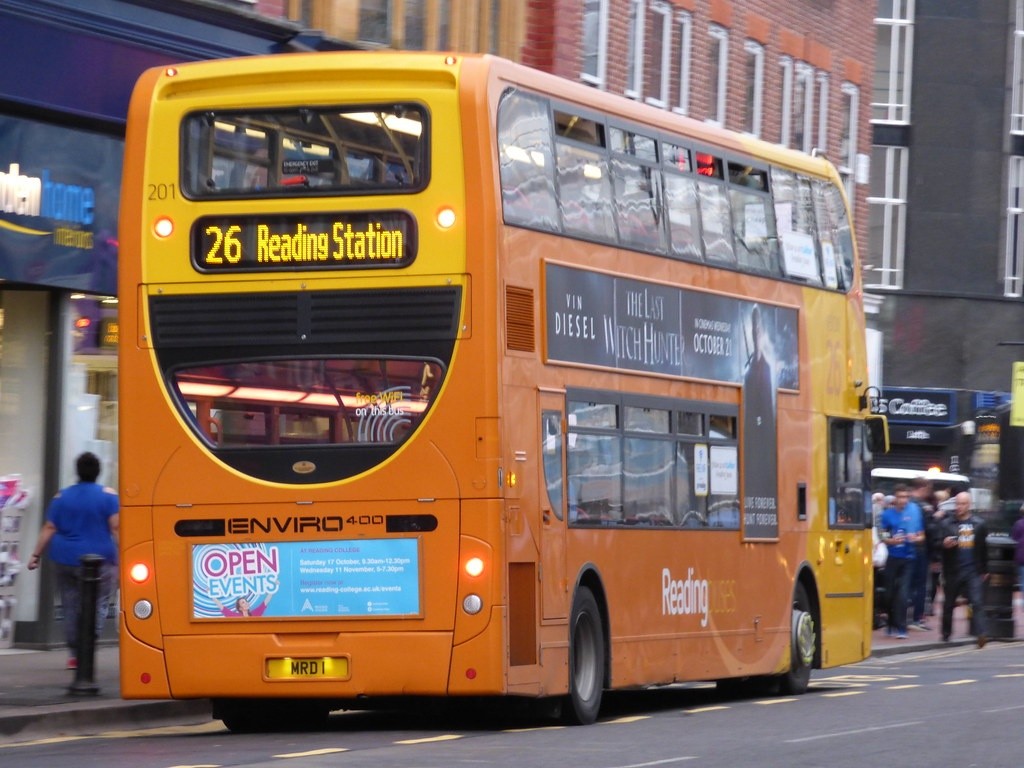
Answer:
[745,304,774,414]
[28,452,119,669]
[873,476,967,638]
[1010,504,1024,611]
[934,491,990,648]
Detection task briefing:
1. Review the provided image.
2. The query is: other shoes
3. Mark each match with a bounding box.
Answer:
[941,635,947,641]
[886,628,892,637]
[67,656,78,671]
[896,629,907,638]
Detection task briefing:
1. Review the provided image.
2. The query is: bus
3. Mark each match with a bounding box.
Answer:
[110,43,896,733]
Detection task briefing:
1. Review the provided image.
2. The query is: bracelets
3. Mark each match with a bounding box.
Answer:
[32,555,39,557]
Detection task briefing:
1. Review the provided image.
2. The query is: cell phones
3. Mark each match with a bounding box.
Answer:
[951,536,959,542]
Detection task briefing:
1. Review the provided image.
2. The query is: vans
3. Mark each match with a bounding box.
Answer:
[872,467,970,500]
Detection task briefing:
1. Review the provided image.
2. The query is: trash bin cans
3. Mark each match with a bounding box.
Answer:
[968,532,1020,639]
